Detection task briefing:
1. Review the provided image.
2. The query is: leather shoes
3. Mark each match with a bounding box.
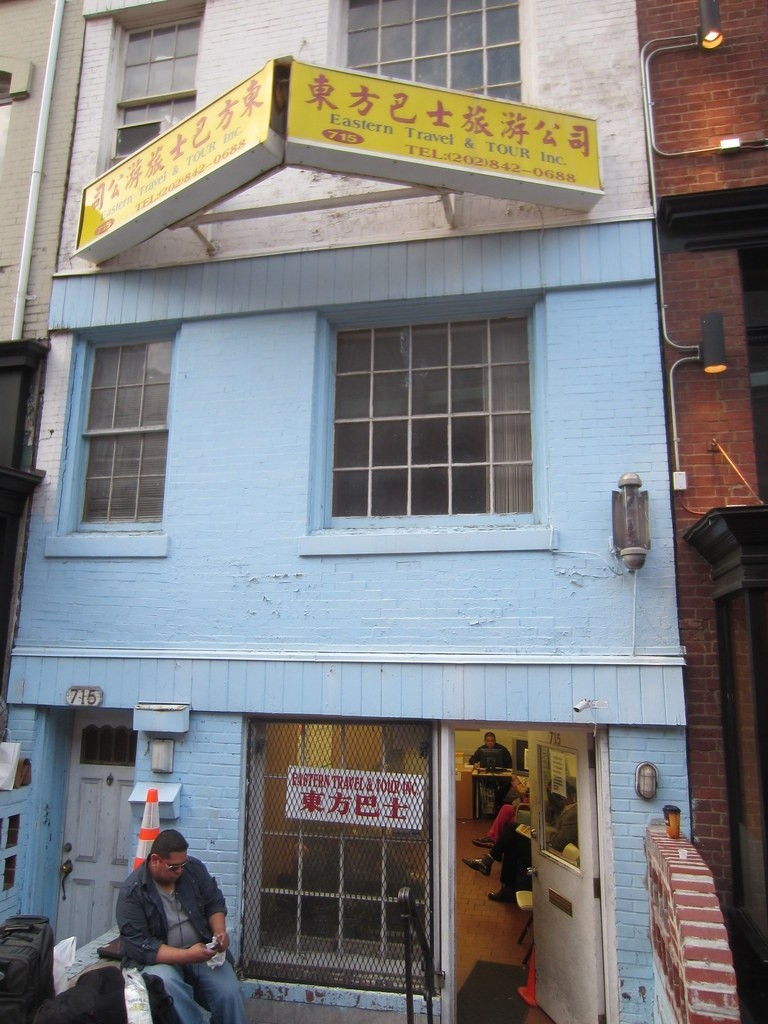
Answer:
[487,891,515,903]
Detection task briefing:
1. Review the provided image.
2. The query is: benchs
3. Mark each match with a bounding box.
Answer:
[67,925,120,989]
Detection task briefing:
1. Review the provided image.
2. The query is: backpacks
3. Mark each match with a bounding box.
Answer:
[32,965,180,1024]
[1,914,57,1024]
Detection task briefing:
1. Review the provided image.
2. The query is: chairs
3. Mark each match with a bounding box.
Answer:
[516,843,580,963]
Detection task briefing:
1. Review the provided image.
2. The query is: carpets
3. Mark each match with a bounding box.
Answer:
[456,959,530,1024]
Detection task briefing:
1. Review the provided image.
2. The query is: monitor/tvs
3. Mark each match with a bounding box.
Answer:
[480,749,503,773]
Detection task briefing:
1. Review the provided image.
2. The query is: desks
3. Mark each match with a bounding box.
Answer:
[472,768,512,819]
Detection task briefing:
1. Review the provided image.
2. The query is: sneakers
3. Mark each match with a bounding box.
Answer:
[462,857,491,876]
[472,834,495,848]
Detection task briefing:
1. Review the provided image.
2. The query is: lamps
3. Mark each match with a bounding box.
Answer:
[611,473,652,570]
[151,738,174,773]
[699,0,724,51]
[635,760,659,801]
[699,310,727,375]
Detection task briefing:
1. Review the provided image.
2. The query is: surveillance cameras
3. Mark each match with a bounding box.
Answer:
[573,701,588,712]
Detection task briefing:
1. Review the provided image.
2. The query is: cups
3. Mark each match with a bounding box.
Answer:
[662,805,681,840]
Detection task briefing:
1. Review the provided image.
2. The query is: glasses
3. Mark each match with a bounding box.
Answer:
[152,854,189,871]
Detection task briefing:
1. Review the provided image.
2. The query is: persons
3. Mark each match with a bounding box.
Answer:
[115,829,248,1024]
[469,732,512,818]
[472,785,529,849]
[462,778,578,902]
